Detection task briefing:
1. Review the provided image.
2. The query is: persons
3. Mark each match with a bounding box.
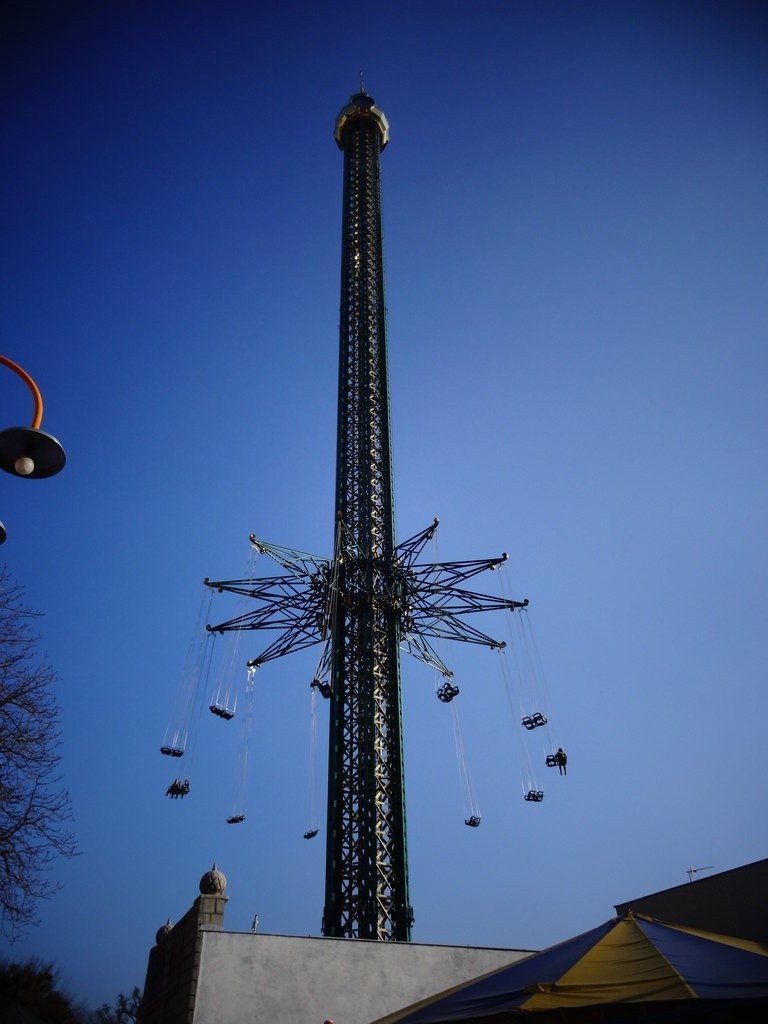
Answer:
[165,778,189,799]
[554,748,567,775]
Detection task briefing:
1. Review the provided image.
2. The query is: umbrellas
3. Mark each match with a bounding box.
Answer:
[370,907,768,1024]
